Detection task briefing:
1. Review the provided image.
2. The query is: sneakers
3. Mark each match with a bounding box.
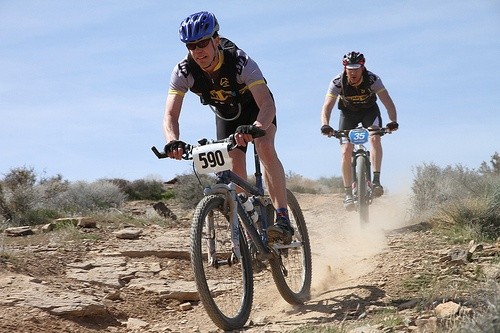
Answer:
[372,183,383,197]
[344,199,354,211]
[267,222,295,245]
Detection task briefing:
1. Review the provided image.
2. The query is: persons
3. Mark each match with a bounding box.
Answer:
[320,51,399,212]
[162,11,293,273]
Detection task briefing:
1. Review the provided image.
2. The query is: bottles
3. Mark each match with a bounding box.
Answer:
[237,192,259,224]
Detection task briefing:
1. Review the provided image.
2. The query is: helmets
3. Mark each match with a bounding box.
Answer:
[342,51,365,69]
[178,11,219,44]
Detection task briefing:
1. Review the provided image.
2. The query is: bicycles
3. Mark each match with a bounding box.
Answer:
[152,125,312,333]
[323,124,398,225]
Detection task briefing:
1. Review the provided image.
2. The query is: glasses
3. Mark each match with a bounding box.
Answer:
[186,38,211,50]
